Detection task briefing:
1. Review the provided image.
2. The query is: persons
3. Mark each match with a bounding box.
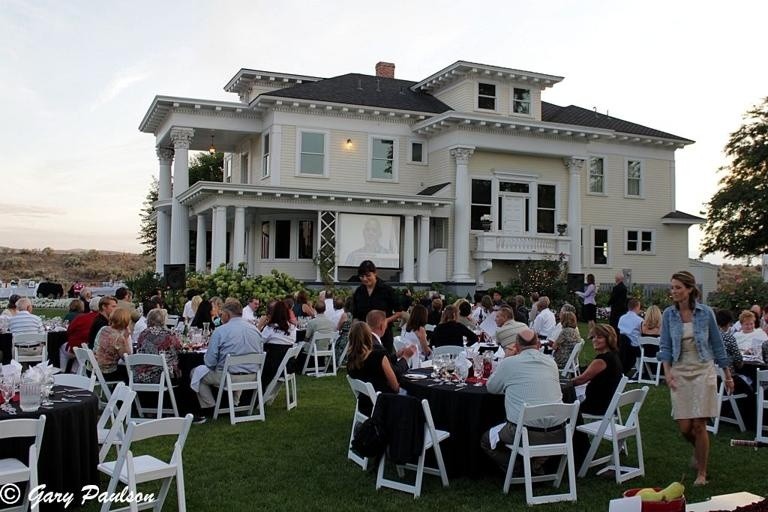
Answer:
[344,217,394,268]
[655,270,735,489]
[561,322,624,470]
[605,272,629,332]
[350,259,407,355]
[0,280,768,426]
[572,272,598,341]
[479,328,570,487]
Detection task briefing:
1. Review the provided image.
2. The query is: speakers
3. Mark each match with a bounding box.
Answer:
[164,264,186,290]
[567,273,584,293]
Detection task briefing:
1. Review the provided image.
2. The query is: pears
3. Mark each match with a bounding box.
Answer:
[635,472,687,502]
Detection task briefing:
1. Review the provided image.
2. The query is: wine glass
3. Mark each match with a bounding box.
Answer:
[432,352,492,387]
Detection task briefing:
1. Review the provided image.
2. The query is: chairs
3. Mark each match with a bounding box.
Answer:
[1,286,768,512]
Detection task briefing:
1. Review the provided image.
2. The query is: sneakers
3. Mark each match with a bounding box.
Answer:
[192,416,210,425]
[690,453,711,488]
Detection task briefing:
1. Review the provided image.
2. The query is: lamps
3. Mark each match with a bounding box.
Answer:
[209,135,216,155]
[346,139,352,149]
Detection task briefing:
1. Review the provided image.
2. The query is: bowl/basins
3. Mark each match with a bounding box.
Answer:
[623,488,685,511]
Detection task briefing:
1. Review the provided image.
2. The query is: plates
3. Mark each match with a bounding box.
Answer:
[404,373,427,381]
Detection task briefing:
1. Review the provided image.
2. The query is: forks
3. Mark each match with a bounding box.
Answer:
[49,387,92,403]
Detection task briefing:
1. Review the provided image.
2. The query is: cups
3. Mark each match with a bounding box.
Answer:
[19,383,40,412]
[173,322,211,341]
